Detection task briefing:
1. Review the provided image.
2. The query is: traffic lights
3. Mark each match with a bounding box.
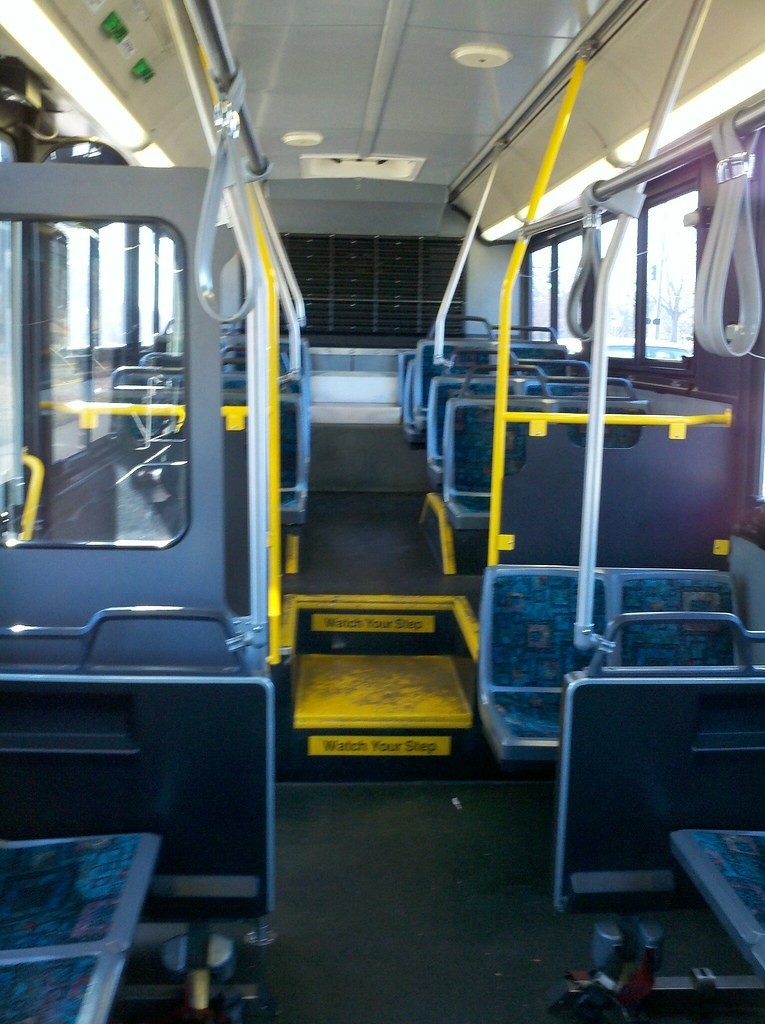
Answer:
[651,264,657,280]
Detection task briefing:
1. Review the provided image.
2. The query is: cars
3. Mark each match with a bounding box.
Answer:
[556,338,693,360]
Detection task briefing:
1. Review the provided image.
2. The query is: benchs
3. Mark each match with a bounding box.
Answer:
[1,316,763,1024]
